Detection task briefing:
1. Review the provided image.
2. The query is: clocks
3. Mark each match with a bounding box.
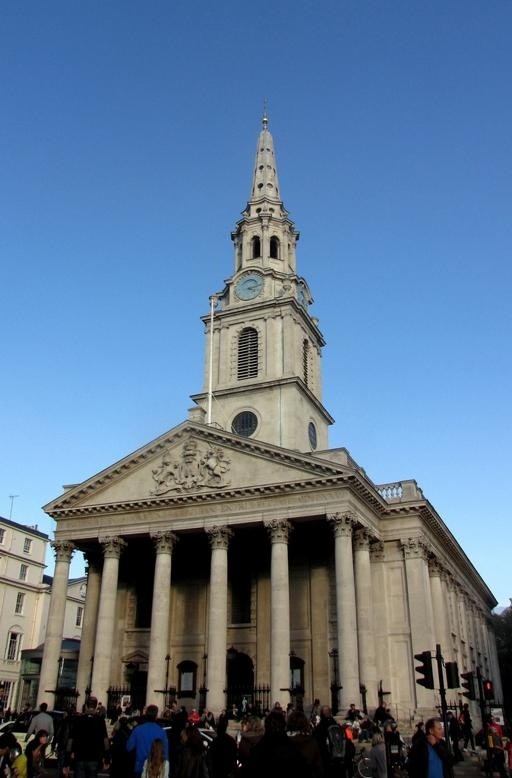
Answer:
[234,273,264,300]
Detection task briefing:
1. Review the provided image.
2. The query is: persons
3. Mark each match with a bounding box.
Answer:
[1,694,510,778]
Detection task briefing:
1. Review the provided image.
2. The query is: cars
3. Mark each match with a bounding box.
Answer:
[0,719,60,760]
[158,725,217,748]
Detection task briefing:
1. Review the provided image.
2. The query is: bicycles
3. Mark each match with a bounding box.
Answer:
[355,746,372,777]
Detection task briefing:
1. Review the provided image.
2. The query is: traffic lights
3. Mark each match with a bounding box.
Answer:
[482,677,496,700]
[413,647,436,690]
[460,669,477,701]
